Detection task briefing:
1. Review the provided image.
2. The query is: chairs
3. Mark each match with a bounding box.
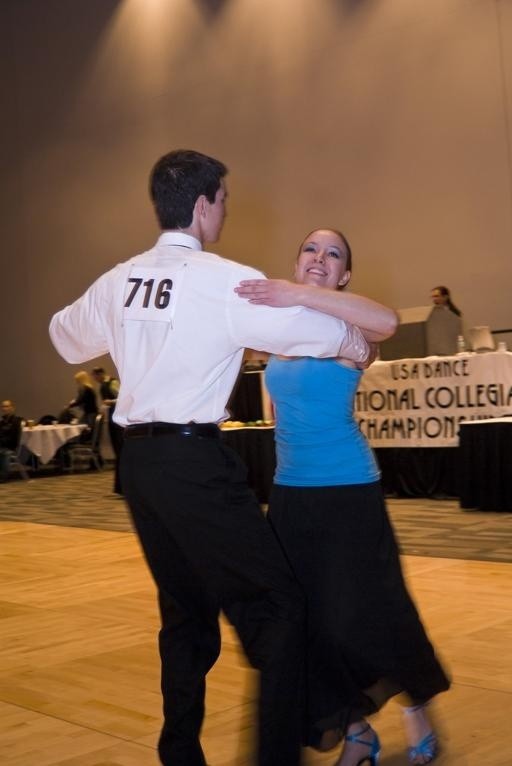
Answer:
[1,418,28,480]
[66,412,104,474]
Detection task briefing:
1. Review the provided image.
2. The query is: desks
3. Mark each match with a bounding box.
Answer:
[21,423,89,474]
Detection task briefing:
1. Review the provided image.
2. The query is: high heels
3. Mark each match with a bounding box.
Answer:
[399,702,441,765]
[335,721,383,766]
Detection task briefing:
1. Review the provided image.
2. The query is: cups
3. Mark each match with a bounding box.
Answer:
[27,420,34,430]
[499,341,506,353]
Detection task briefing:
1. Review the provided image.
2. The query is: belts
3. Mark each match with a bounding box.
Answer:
[117,424,219,440]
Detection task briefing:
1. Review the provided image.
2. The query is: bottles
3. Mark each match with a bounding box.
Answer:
[458,336,464,353]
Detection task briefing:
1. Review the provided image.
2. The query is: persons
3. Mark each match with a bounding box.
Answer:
[92,366,123,467]
[431,283,465,318]
[63,370,103,471]
[241,227,455,765]
[1,398,21,453]
[47,150,381,766]
